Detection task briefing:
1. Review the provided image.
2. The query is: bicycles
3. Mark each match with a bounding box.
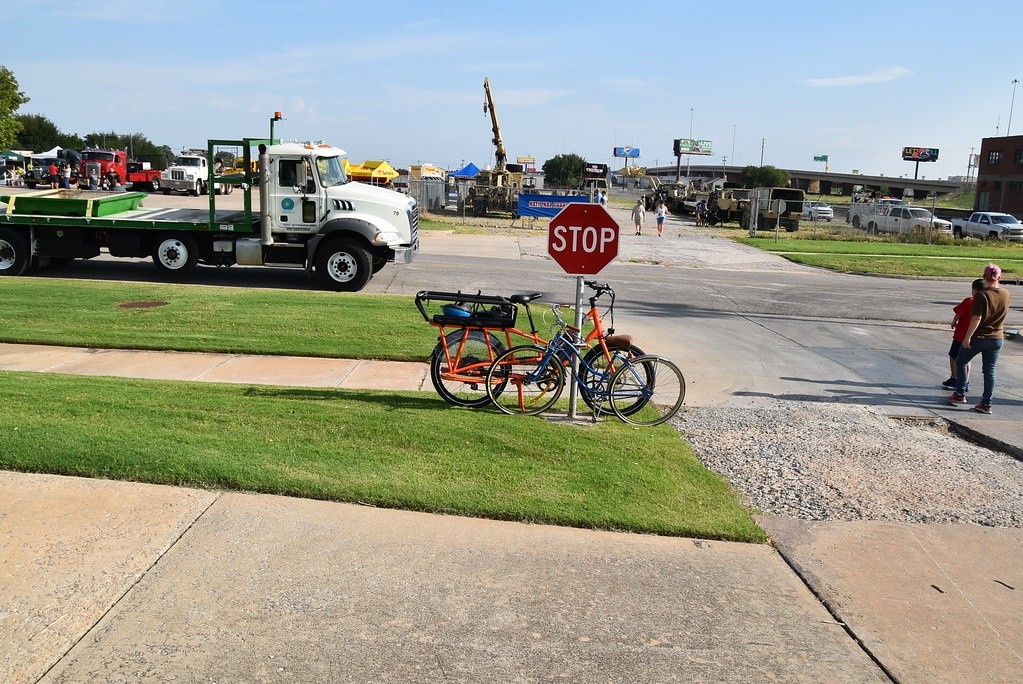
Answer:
[415,279,690,424]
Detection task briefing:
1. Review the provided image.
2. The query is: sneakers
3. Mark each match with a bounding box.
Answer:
[965,382,970,392]
[942,376,957,387]
[946,392,967,403]
[975,402,992,414]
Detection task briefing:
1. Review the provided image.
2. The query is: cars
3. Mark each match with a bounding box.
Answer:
[581,178,610,202]
[844,198,907,229]
[21,158,74,189]
[801,200,833,220]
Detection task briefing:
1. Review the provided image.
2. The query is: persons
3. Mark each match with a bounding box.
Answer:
[7,161,127,192]
[565,190,581,196]
[631,199,646,236]
[594,187,609,207]
[942,279,988,392]
[695,199,723,227]
[641,192,667,210]
[947,264,1011,414]
[655,200,671,237]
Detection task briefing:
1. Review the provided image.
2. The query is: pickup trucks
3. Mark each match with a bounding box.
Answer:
[952,212,1023,243]
[860,204,953,239]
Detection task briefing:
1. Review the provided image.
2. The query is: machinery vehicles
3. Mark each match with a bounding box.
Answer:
[716,186,805,232]
[461,75,535,220]
[616,166,692,212]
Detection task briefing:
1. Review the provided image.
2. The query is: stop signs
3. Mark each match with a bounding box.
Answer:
[548,203,622,274]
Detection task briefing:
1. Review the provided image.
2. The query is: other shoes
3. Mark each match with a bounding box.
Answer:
[636,232,641,235]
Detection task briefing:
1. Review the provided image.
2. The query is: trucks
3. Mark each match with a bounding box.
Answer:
[0,139,418,292]
[159,153,255,196]
[75,146,162,190]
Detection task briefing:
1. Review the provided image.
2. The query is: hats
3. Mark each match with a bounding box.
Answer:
[660,200,665,204]
[638,200,642,203]
